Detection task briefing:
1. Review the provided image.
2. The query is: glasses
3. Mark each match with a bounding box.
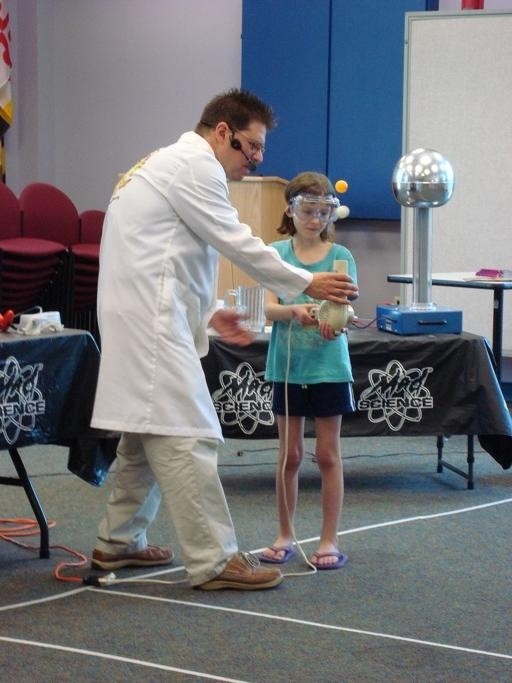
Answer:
[287,193,340,224]
[235,127,266,154]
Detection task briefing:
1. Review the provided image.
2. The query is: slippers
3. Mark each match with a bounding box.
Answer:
[310,552,347,570]
[260,545,296,563]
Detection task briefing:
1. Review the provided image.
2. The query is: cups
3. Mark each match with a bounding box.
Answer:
[225,284,267,334]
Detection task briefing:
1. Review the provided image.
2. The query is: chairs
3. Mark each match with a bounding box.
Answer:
[1,181,104,330]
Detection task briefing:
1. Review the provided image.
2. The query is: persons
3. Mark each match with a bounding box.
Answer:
[90,91,359,590]
[259,171,347,569]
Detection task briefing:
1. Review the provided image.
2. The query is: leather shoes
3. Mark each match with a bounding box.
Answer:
[91,543,175,570]
[200,551,283,592]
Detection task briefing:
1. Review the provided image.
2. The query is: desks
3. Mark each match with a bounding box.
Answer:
[199,322,512,488]
[386,271,512,403]
[1,323,122,559]
